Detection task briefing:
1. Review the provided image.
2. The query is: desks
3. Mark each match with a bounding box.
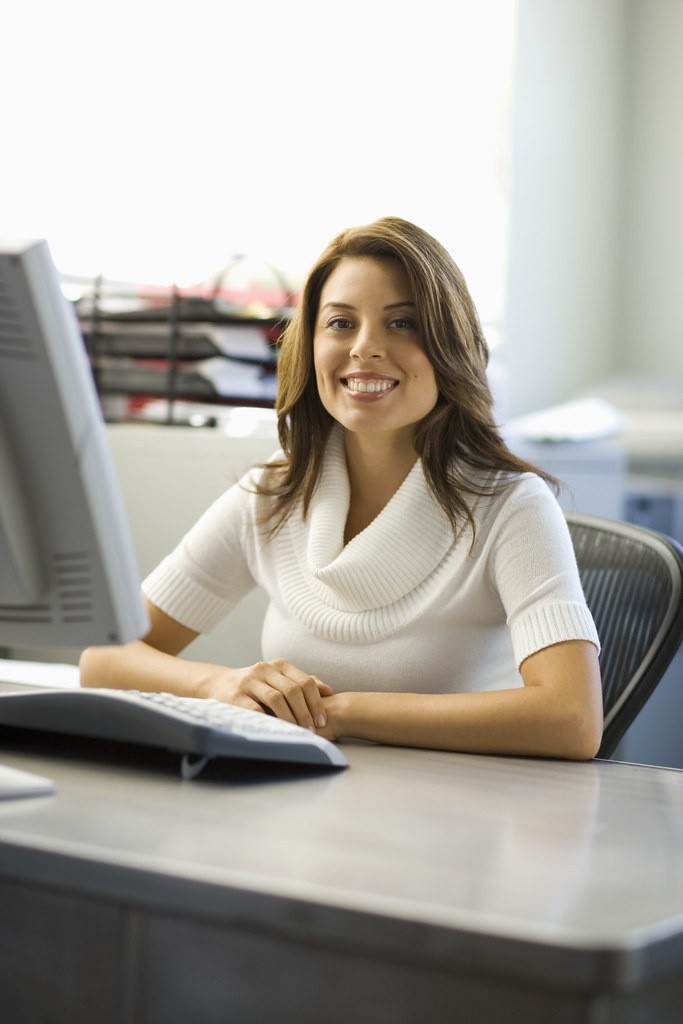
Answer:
[0,680,683,1024]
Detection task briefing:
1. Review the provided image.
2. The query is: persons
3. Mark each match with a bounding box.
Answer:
[78,217,605,762]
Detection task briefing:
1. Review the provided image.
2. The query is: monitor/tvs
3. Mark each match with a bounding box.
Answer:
[0,237,151,797]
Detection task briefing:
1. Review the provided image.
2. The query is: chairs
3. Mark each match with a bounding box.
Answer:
[564,510,683,760]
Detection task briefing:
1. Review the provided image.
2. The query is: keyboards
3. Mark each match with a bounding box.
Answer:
[0,687,351,769]
